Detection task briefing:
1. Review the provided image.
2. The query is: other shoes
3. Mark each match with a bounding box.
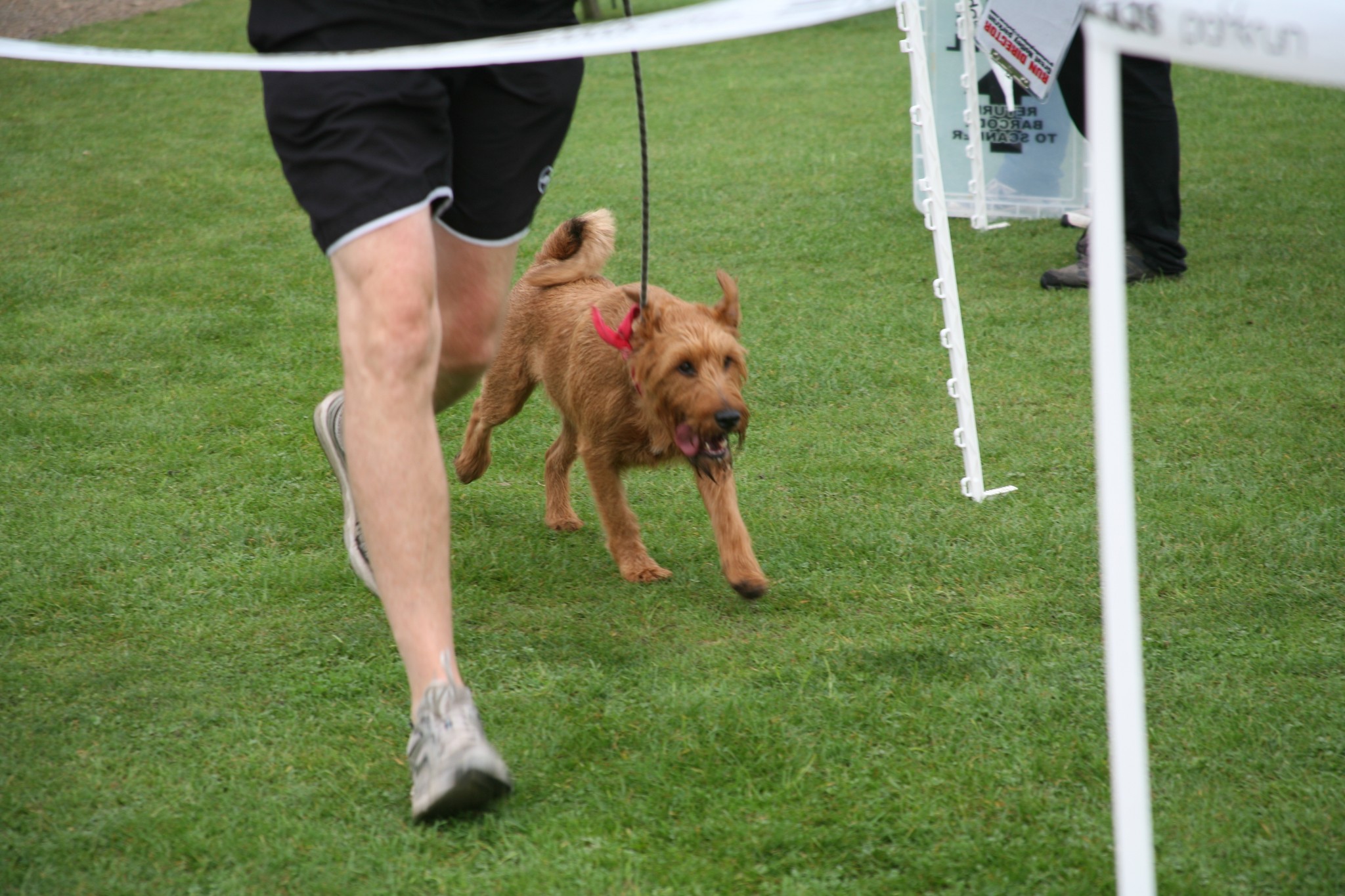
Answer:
[1062,212,1091,229]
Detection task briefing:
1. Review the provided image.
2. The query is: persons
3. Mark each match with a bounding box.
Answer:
[247,0,589,816]
[1038,0,1189,288]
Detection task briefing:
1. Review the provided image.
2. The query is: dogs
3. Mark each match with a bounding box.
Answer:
[453,206,768,600]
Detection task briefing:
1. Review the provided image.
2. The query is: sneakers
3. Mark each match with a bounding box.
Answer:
[1076,228,1088,256]
[1040,250,1154,291]
[406,654,511,824]
[315,390,382,594]
